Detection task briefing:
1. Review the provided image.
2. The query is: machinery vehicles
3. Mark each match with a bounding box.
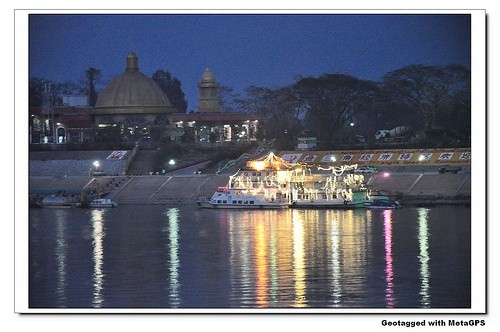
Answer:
[375,126,411,142]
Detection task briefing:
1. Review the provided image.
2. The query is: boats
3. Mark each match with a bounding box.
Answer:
[34,196,82,209]
[195,152,378,208]
[362,196,399,209]
[87,199,117,208]
